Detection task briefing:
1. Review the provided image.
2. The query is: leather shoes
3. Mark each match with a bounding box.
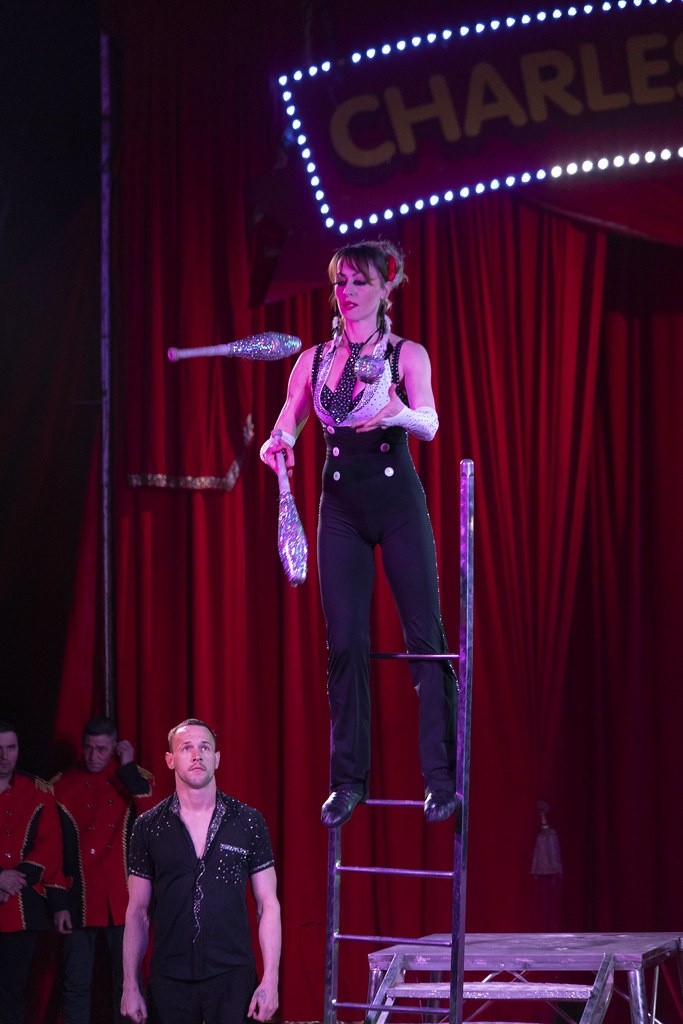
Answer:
[424,792,456,822]
[329,326,381,426]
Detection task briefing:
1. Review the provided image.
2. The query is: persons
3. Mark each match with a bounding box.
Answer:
[0,717,163,1024]
[121,719,282,1024]
[261,241,457,827]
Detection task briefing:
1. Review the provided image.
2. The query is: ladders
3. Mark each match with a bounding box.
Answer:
[321,456,475,1024]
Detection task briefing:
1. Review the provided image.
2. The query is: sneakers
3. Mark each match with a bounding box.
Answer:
[321,790,369,827]
